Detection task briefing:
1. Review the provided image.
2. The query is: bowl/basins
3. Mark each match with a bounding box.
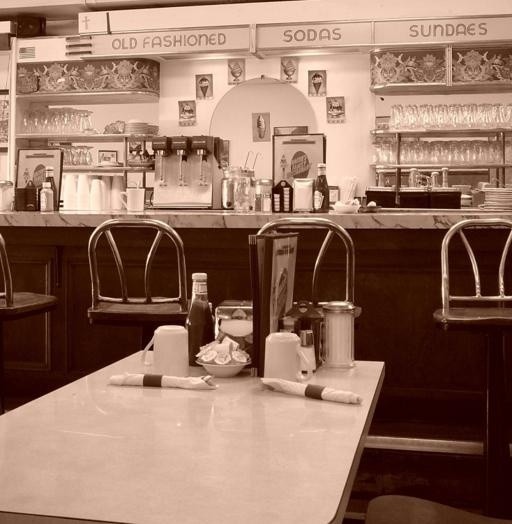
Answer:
[452,183,473,207]
[330,203,362,214]
[193,348,254,378]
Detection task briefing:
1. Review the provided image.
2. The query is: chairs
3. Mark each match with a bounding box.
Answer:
[253,218,362,331]
[432,219,512,521]
[0,234,59,414]
[368,494,507,523]
[86,219,192,350]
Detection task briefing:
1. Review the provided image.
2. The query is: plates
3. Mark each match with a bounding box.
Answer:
[480,187,512,212]
[360,206,382,213]
[123,120,159,135]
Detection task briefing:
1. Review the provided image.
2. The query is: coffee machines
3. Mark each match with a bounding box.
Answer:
[151,135,228,209]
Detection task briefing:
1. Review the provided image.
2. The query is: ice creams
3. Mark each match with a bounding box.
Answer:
[284,58,296,81]
[311,72,323,96]
[199,75,210,97]
[178,103,195,118]
[230,62,241,83]
[326,99,346,117]
[257,115,265,138]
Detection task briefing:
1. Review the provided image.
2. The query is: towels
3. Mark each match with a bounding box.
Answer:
[260,376,364,406]
[108,371,219,391]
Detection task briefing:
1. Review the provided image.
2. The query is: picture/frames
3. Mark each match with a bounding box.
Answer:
[0,89,10,152]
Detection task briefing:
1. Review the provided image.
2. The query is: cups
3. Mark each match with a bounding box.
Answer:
[62,172,146,213]
[55,143,96,166]
[262,331,314,386]
[20,107,97,135]
[140,323,190,380]
[370,139,512,166]
[386,103,512,130]
[471,189,486,207]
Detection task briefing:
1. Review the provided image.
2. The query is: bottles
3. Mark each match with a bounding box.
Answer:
[313,163,331,213]
[0,166,57,212]
[219,166,273,214]
[282,298,355,369]
[182,271,215,367]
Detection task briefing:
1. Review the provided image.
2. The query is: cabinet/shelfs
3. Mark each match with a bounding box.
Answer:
[9,31,161,211]
[366,43,512,212]
